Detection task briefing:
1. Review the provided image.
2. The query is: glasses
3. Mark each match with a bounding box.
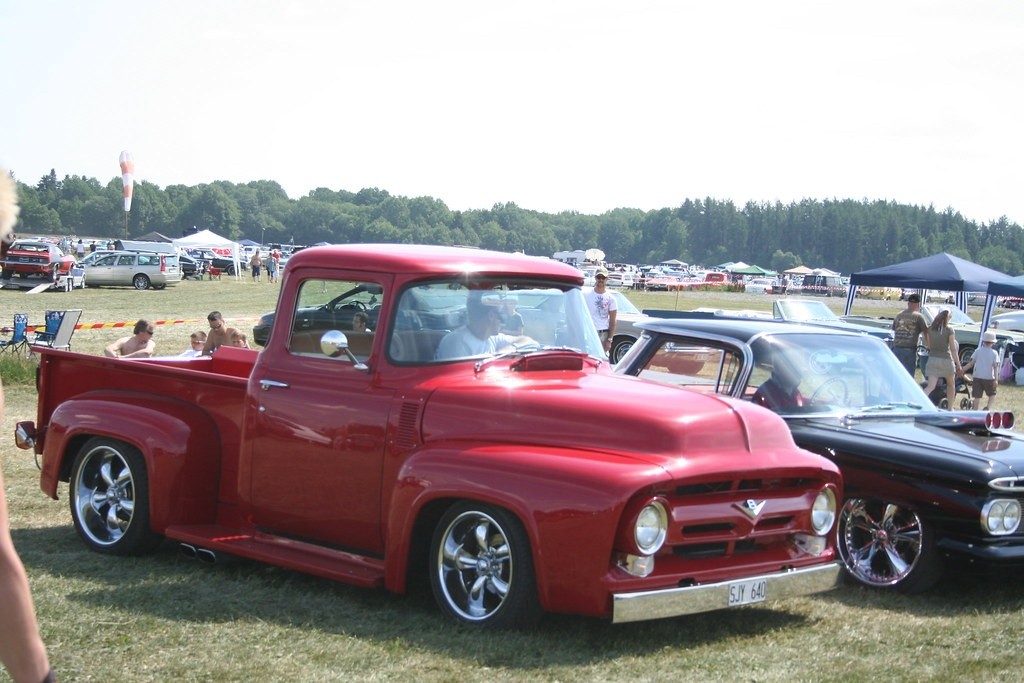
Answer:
[210,321,223,329]
[144,328,153,336]
[596,275,606,280]
[190,340,206,345]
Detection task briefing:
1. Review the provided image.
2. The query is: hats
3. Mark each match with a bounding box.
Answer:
[595,267,608,278]
[982,331,997,343]
[938,306,952,318]
[908,294,921,302]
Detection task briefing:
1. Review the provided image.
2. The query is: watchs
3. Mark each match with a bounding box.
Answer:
[511,342,518,352]
[607,338,613,343]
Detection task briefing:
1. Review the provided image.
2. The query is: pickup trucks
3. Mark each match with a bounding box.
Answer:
[13,243,844,635]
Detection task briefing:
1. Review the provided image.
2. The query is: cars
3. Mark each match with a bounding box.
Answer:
[584,288,707,375]
[179,243,301,279]
[898,303,1024,385]
[53,255,86,291]
[712,299,922,368]
[79,249,150,269]
[899,287,1024,310]
[577,262,871,298]
[607,316,1024,594]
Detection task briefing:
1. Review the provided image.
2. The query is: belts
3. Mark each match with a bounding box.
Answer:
[597,328,609,333]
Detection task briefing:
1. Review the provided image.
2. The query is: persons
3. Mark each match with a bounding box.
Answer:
[251,248,280,283]
[352,312,371,332]
[892,294,1000,411]
[781,274,787,295]
[61,237,113,259]
[437,293,539,361]
[0,468,58,683]
[582,266,617,364]
[1001,299,1024,310]
[753,353,805,413]
[104,311,250,359]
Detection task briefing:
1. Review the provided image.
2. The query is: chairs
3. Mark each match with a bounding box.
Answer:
[0,310,82,362]
[291,330,452,362]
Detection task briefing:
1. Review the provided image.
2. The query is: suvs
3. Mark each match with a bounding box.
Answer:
[2,241,77,283]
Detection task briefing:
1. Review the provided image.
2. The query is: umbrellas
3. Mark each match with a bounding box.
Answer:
[585,248,605,261]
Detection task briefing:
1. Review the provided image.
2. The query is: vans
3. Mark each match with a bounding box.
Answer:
[83,252,181,291]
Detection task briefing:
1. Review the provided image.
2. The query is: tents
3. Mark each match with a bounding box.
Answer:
[716,261,841,296]
[659,258,688,268]
[979,275,1024,349]
[131,229,263,280]
[845,252,1013,316]
